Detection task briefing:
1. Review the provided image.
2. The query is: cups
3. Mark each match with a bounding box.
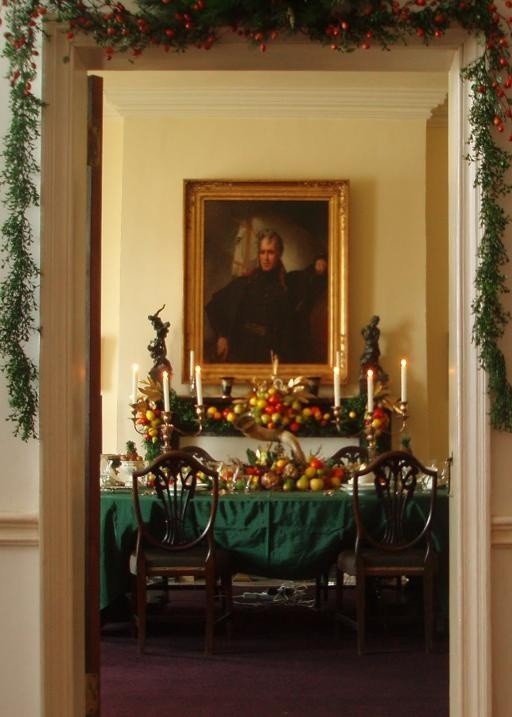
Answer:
[307,376,321,399]
[219,377,234,399]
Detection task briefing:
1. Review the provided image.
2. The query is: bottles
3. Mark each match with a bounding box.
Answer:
[119,461,145,488]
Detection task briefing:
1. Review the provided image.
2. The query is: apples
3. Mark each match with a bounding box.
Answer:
[135,387,389,490]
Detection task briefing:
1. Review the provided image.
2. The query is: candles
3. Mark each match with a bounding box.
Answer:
[367,370,374,412]
[401,360,407,402]
[334,368,340,406]
[195,366,202,405]
[164,372,170,412]
[132,365,137,403]
[189,351,194,378]
[336,352,341,368]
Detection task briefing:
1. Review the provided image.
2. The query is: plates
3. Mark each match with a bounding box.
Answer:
[339,483,387,496]
[166,484,208,497]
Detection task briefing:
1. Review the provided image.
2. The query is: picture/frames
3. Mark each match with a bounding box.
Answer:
[182,177,349,387]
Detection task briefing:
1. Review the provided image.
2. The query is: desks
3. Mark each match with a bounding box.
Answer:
[101,489,449,625]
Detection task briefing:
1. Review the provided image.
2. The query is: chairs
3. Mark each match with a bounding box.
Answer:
[316,446,368,607]
[182,446,216,461]
[132,452,233,658]
[336,451,438,657]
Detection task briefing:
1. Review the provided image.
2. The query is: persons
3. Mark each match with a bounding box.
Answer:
[147,321,172,364]
[358,313,384,369]
[203,227,329,365]
[147,303,170,336]
